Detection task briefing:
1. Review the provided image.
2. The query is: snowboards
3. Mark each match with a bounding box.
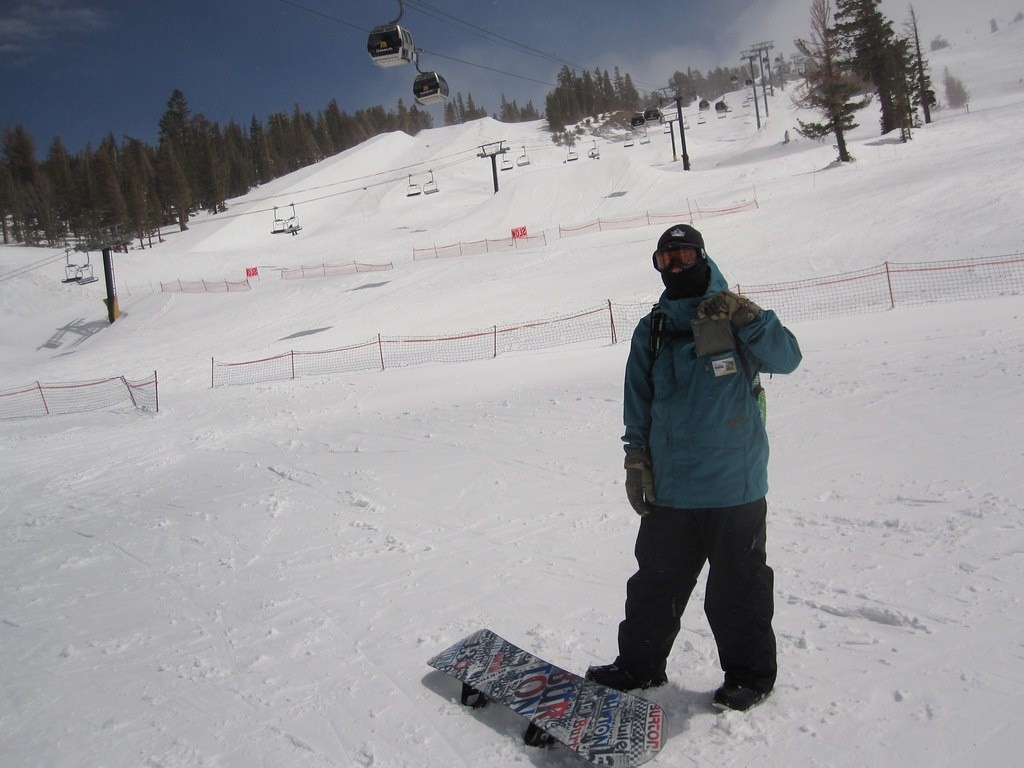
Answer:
[427,625,667,768]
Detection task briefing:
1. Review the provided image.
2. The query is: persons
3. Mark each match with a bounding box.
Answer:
[586,224,802,712]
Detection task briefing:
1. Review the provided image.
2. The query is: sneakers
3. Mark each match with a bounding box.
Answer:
[711,682,773,713]
[584,660,669,692]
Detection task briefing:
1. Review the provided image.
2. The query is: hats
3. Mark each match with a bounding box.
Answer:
[658,223,705,252]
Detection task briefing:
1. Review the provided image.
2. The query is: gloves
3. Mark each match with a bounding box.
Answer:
[697,290,762,328]
[623,446,657,518]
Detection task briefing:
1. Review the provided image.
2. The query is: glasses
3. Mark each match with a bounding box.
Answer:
[653,242,705,274]
